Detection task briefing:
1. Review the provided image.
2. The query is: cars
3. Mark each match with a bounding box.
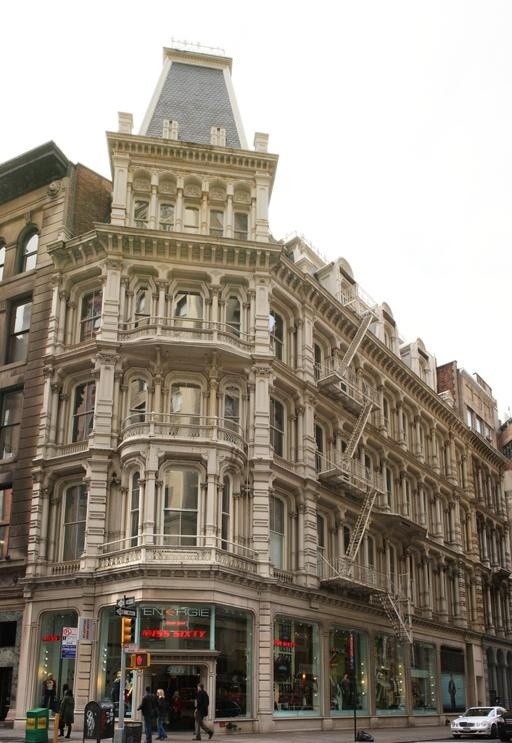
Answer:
[450,705,507,738]
[498,708,512,741]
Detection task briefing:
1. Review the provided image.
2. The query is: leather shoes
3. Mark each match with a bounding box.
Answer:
[192,737,201,740]
[209,731,213,739]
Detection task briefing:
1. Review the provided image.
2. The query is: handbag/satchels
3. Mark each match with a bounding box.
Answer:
[194,709,198,720]
[59,715,64,729]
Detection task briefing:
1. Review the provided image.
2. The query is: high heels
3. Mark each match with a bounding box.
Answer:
[156,735,162,740]
[161,736,168,740]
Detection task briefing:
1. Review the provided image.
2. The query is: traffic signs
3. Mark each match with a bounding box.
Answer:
[116,596,135,608]
[114,607,136,617]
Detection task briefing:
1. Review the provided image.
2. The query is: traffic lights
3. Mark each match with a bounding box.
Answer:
[123,618,134,644]
[130,652,152,670]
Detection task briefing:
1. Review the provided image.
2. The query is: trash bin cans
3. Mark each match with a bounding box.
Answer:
[125,721,142,743]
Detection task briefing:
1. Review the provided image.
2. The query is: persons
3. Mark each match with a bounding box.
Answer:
[340,672,351,710]
[448,673,457,712]
[57,679,214,743]
[495,697,503,707]
[376,666,388,703]
[42,673,56,710]
[111,671,131,694]
